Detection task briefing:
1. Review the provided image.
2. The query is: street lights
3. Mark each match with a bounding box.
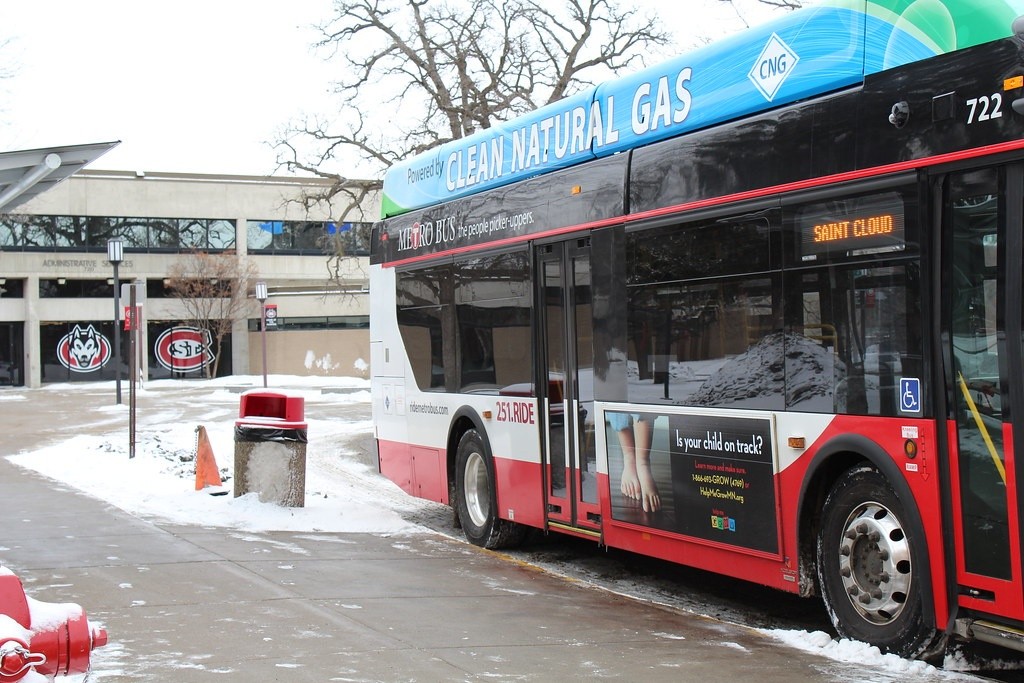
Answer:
[108,238,125,404]
[256,282,268,388]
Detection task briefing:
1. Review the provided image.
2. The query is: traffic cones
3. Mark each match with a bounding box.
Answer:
[196,425,222,491]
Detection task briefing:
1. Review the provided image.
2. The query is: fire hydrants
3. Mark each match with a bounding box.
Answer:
[0,566,108,683]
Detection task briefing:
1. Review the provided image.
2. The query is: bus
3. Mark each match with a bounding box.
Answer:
[368,1,1023,667]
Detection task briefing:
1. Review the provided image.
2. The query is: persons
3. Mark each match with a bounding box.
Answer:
[607,411,662,513]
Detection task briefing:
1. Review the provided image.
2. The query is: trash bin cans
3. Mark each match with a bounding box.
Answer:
[234,390,308,508]
